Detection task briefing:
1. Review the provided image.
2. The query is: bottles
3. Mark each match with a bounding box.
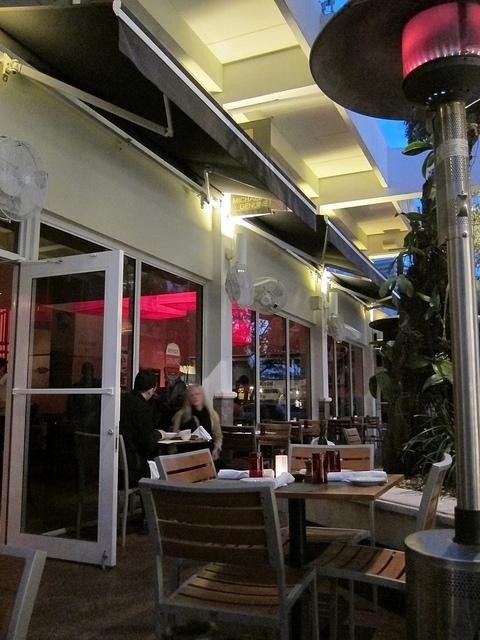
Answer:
[248,452,263,478]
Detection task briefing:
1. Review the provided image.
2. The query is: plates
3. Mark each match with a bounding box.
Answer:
[352,481,382,487]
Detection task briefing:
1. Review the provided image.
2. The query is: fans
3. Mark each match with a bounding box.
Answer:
[328,313,346,342]
[225,263,254,308]
[0,134,49,223]
[252,280,288,316]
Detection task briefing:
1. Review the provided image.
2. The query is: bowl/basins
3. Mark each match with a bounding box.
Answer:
[166,433,179,439]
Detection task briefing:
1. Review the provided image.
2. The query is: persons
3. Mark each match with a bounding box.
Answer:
[0,358,8,418]
[232,375,256,427]
[66,364,101,426]
[91,371,163,534]
[171,384,223,474]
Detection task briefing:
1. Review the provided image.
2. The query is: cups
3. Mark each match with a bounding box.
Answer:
[180,429,191,440]
[326,450,341,471]
[313,453,328,483]
[260,425,265,435]
[351,416,355,423]
[304,420,309,428]
